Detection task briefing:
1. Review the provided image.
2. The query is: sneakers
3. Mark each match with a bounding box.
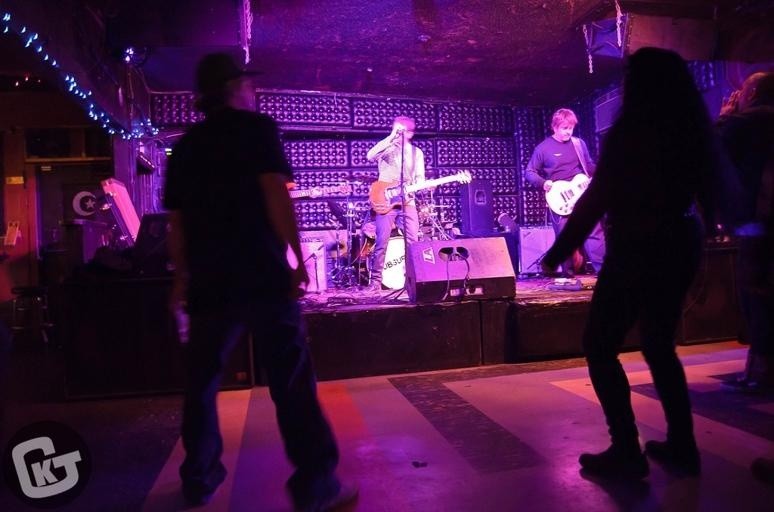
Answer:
[182,461,227,505]
[752,457,774,485]
[727,375,774,393]
[646,430,701,473]
[295,482,360,512]
[579,443,650,478]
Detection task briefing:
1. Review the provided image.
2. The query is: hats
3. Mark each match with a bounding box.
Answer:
[196,51,266,91]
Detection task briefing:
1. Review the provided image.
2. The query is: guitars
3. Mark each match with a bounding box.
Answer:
[369,167,473,216]
[286,181,352,199]
[545,173,594,217]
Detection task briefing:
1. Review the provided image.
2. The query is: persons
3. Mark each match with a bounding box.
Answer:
[160,45,359,511]
[525,108,606,276]
[751,456,774,487]
[361,115,436,296]
[537,46,720,486]
[705,71,773,397]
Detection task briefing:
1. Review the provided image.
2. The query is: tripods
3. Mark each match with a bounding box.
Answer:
[327,212,378,286]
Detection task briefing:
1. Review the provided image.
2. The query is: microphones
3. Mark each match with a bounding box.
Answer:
[396,128,405,135]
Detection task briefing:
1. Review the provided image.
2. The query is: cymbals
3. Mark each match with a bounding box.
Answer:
[343,175,378,182]
[326,200,357,232]
[426,220,458,224]
[422,203,450,209]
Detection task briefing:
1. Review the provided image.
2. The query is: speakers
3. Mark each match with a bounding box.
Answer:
[300,228,376,263]
[517,223,565,274]
[57,219,97,263]
[286,237,328,293]
[403,237,517,303]
[462,179,493,233]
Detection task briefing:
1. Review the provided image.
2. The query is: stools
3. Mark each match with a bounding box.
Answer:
[3,282,53,355]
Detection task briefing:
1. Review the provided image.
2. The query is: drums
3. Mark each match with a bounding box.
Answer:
[366,233,422,291]
[348,232,378,264]
